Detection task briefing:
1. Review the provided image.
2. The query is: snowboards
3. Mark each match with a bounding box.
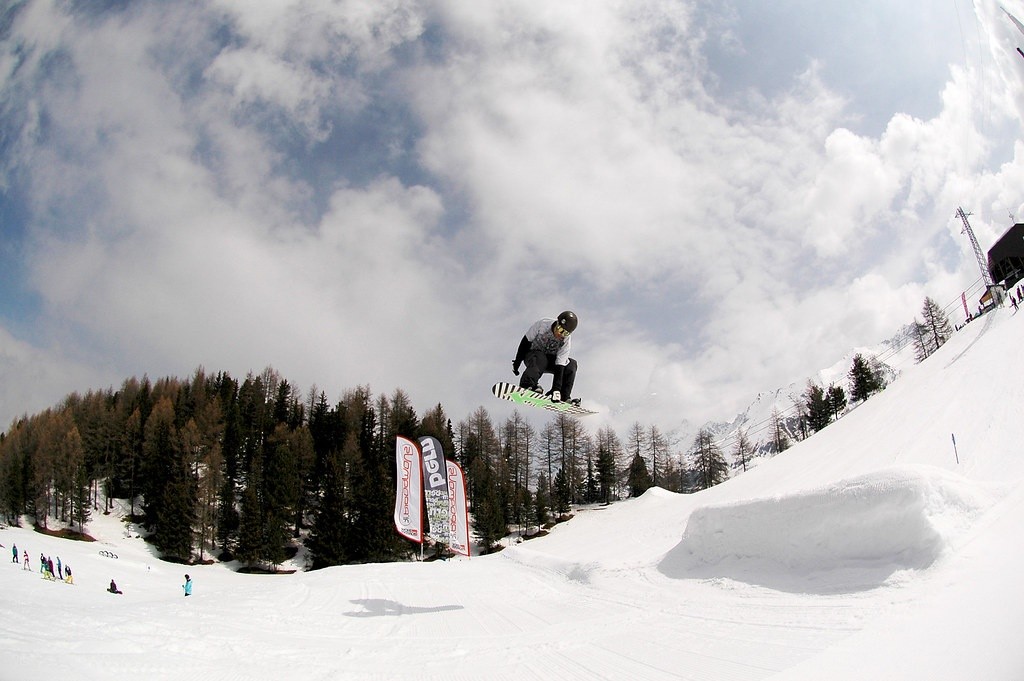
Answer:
[492,381,599,418]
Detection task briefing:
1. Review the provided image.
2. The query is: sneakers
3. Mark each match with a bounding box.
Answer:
[562,397,582,406]
[520,384,543,393]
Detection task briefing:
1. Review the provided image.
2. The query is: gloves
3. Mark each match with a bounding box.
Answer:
[544,388,561,403]
[512,360,521,376]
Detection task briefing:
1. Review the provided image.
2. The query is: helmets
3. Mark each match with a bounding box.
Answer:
[558,311,578,334]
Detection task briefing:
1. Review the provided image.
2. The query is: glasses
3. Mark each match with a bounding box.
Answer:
[557,325,570,336]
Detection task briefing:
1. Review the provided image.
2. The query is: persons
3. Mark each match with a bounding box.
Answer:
[182,575,192,596]
[40,553,73,583]
[1009,285,1024,311]
[13,543,18,563]
[512,310,582,408]
[24,550,31,570]
[110,580,122,594]
